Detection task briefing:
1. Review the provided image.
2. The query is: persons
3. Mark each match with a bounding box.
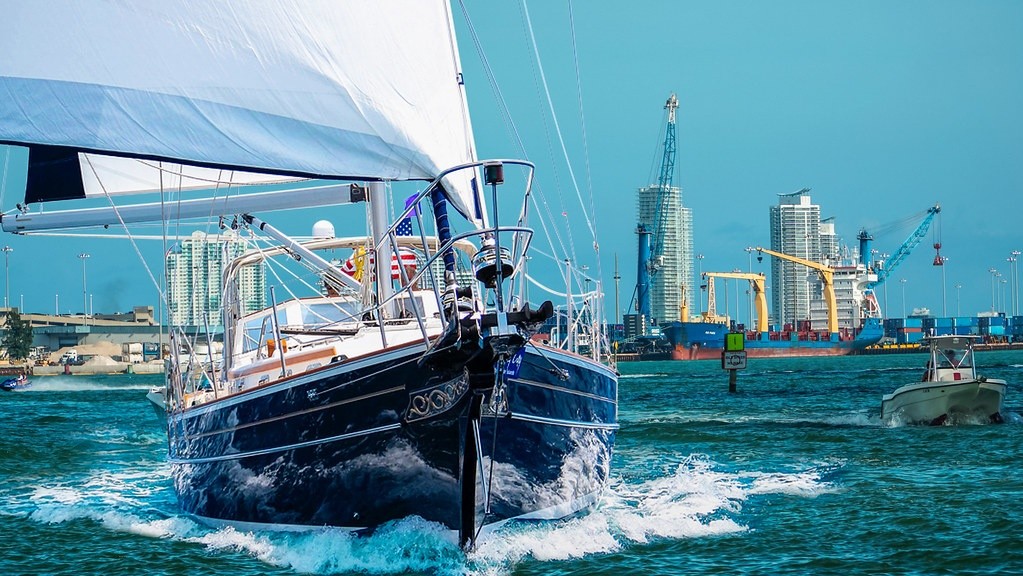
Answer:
[16,371,29,382]
[870,332,1011,348]
[8,351,90,367]
[941,350,966,368]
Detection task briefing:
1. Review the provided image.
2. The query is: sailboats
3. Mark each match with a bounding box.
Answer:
[0,0,619,561]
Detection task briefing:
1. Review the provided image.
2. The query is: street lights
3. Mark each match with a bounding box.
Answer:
[1006,257,1016,316]
[956,284,961,317]
[995,272,1002,313]
[79,253,91,326]
[941,255,949,318]
[900,278,908,328]
[879,253,890,319]
[1011,251,1021,316]
[989,268,996,317]
[1,245,14,328]
[1000,279,1007,314]
[744,247,755,331]
[696,254,704,316]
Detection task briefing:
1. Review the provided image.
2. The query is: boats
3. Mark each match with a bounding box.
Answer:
[658,314,886,362]
[879,328,1008,426]
[0,378,32,390]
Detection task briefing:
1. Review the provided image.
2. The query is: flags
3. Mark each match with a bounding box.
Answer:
[368,251,417,281]
[403,192,423,216]
[342,247,376,284]
[388,216,412,239]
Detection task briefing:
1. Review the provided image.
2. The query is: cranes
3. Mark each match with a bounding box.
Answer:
[700,271,768,341]
[744,246,839,334]
[619,90,679,357]
[860,202,944,318]
